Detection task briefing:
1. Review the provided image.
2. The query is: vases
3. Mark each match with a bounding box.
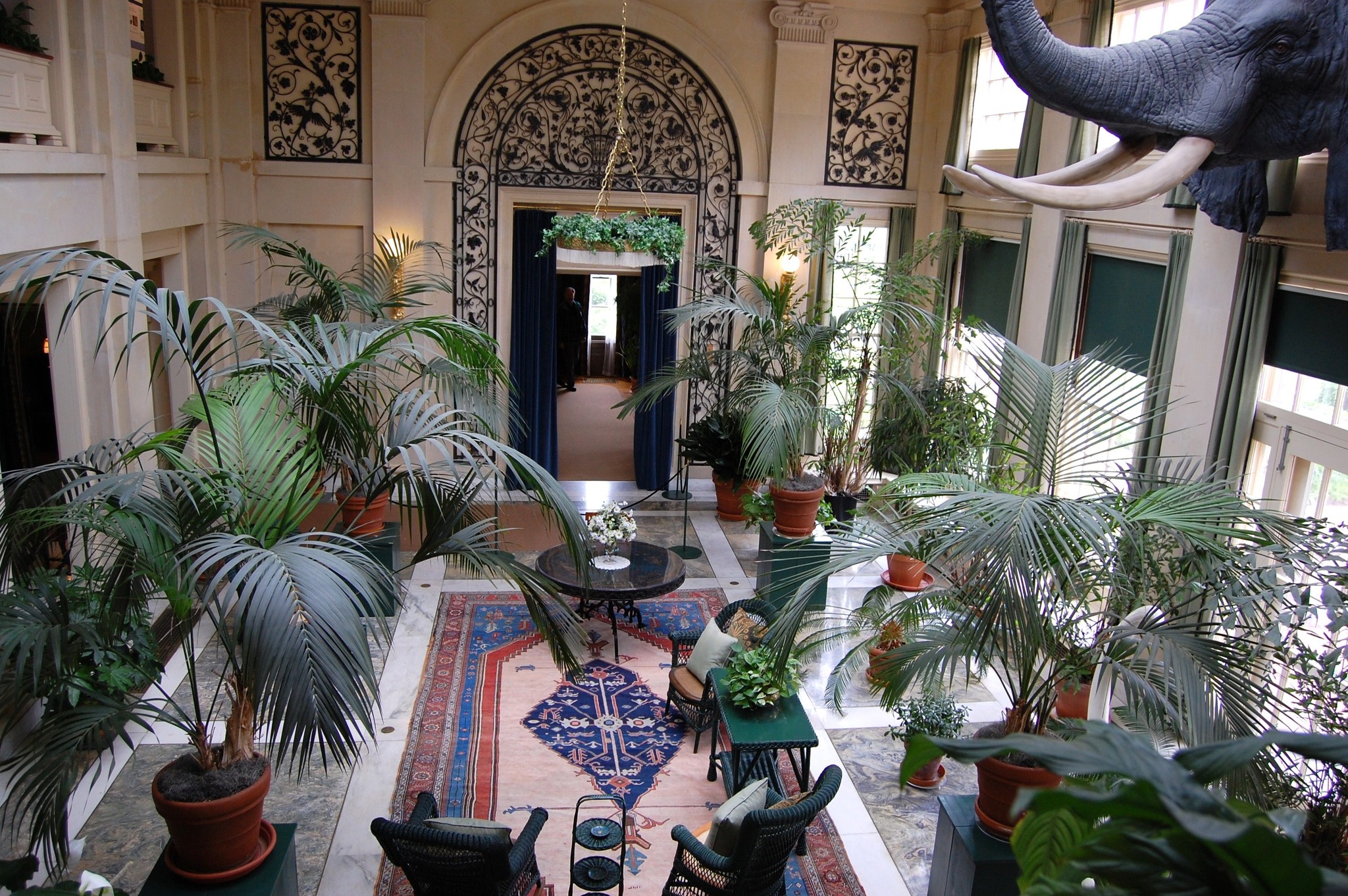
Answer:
[603,540,618,564]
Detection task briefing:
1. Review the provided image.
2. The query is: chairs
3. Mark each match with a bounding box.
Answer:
[669,598,784,753]
[662,763,843,896]
[370,792,548,896]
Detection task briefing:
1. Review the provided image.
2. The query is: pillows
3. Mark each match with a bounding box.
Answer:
[766,791,812,808]
[684,616,738,690]
[704,778,769,856]
[726,607,768,657]
[422,817,514,847]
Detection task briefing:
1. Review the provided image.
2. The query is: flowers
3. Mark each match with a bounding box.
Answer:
[583,499,638,544]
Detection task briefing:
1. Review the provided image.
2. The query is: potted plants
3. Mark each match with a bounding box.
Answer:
[0,220,595,887]
[534,212,1348,896]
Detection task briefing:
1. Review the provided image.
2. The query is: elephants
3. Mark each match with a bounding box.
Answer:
[941,0,1348,252]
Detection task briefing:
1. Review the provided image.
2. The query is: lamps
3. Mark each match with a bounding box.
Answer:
[382,235,404,319]
[782,255,799,322]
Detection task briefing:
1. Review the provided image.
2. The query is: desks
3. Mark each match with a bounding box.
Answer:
[536,539,686,666]
[926,793,1022,896]
[325,520,401,618]
[759,519,834,611]
[138,824,302,896]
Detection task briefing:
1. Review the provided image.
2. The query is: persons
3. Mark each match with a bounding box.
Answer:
[556,287,587,391]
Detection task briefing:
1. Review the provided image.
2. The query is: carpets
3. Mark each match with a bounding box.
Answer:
[369,590,869,896]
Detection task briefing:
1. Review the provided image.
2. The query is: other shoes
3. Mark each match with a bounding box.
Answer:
[567,387,576,392]
[556,383,567,387]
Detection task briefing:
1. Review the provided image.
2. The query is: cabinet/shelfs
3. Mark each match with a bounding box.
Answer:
[567,794,627,896]
[709,667,820,801]
